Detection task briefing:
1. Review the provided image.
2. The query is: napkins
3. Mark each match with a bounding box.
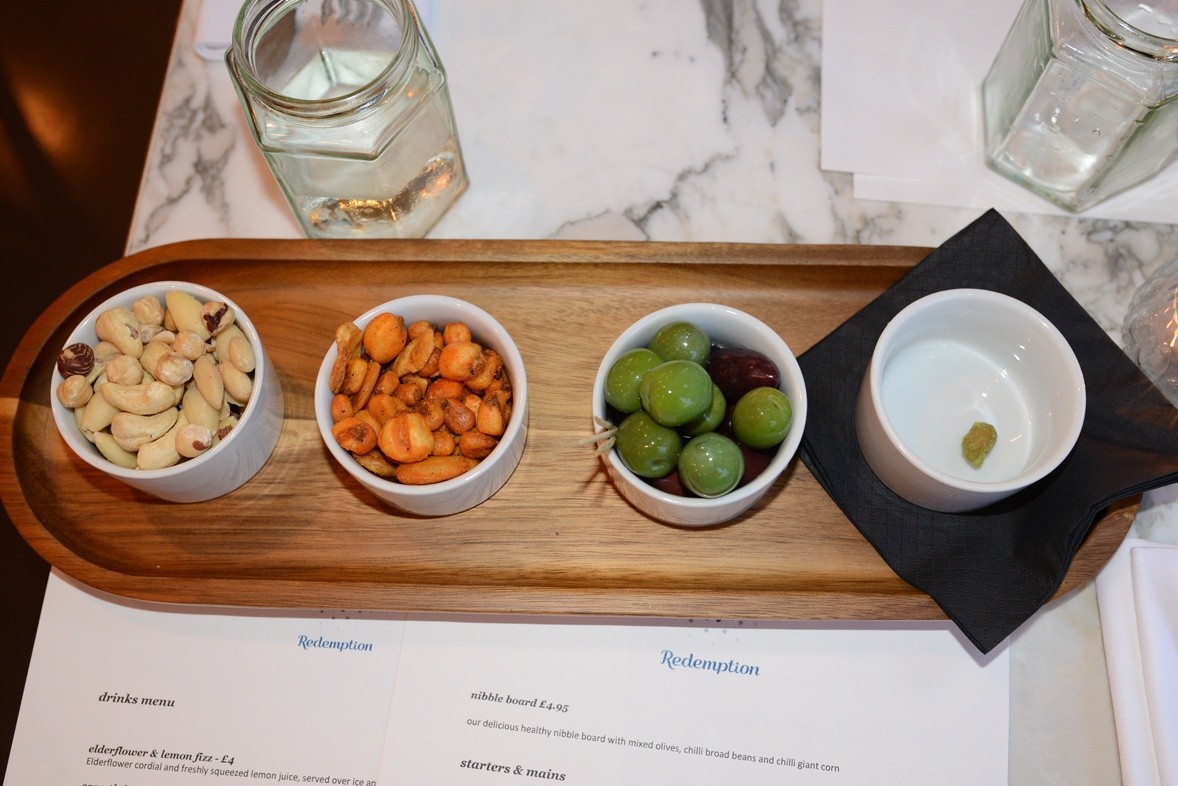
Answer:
[1094,537,1178,786]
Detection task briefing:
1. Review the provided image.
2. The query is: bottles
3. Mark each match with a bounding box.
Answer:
[223,0,470,239]
[982,0,1177,210]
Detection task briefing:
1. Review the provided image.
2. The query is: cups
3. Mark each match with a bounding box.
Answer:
[854,287,1087,514]
[593,302,808,526]
[50,280,285,503]
[315,295,528,516]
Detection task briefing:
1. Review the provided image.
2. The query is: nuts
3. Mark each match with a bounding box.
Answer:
[56,291,256,470]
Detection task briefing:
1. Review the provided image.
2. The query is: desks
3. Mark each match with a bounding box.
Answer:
[127,0,1178,786]
